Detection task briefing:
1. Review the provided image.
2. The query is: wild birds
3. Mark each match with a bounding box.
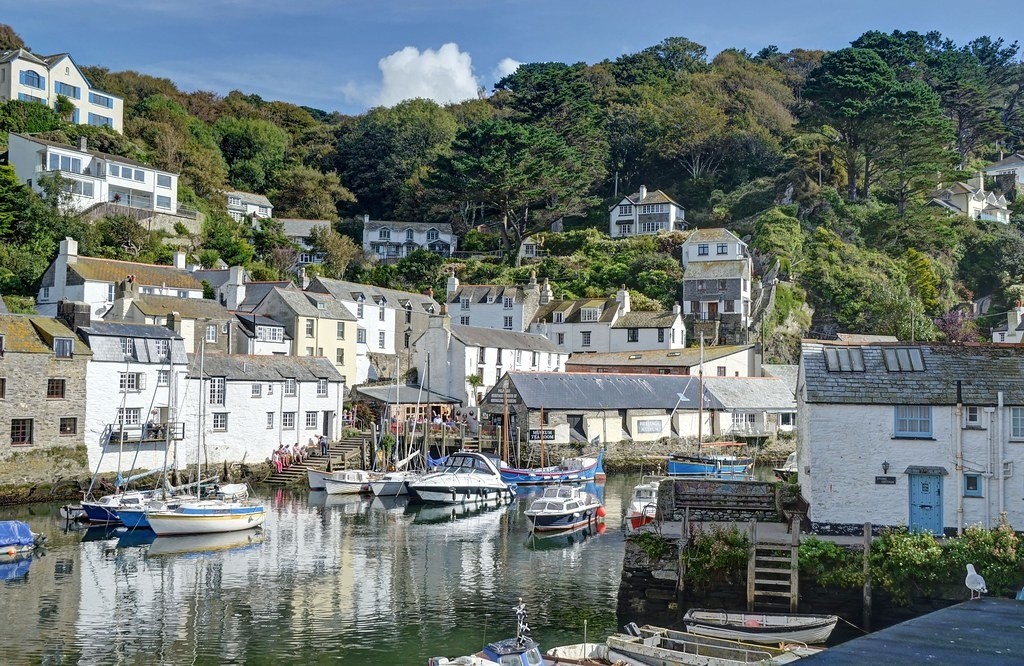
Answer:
[964,563,988,600]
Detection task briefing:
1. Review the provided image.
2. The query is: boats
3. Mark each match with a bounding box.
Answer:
[606,622,828,666]
[624,481,660,532]
[772,451,798,481]
[306,466,333,490]
[545,620,651,666]
[60,505,88,520]
[428,655,500,666]
[0,520,49,582]
[207,483,249,501]
[682,606,838,646]
[407,448,517,504]
[471,597,580,666]
[523,469,605,533]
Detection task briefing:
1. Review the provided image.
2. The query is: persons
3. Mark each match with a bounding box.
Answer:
[410,410,469,431]
[273,434,329,473]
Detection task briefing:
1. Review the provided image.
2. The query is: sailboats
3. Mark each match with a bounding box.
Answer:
[80,348,155,523]
[321,355,400,496]
[115,336,250,529]
[143,335,266,536]
[428,405,607,485]
[643,330,755,478]
[369,352,432,497]
